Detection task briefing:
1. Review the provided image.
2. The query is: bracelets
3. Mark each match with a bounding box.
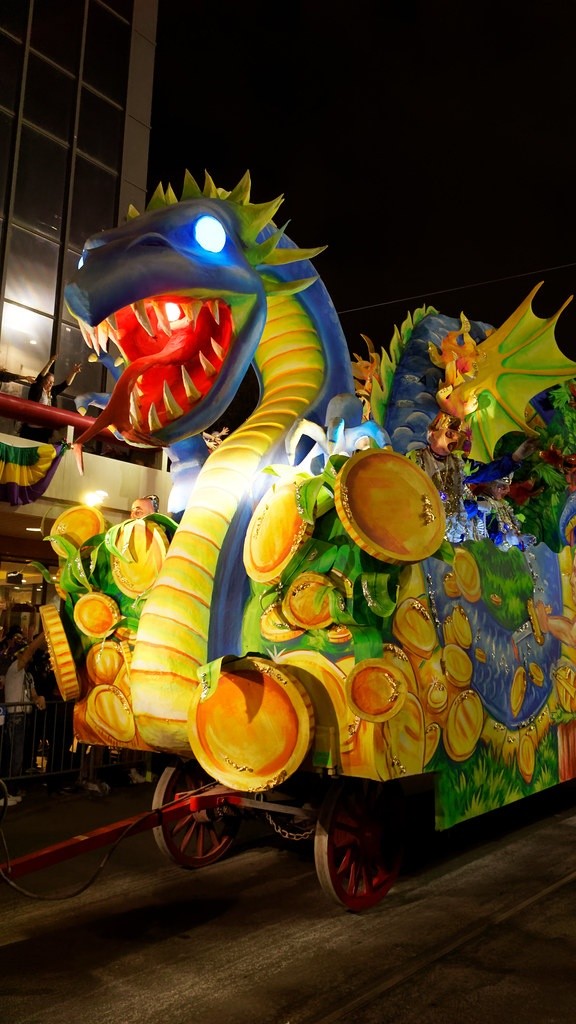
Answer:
[217,434,219,437]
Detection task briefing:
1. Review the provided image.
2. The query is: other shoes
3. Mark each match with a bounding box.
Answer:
[0,794,22,805]
[128,768,145,783]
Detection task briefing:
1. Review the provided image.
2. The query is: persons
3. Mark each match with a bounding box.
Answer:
[202,426,229,450]
[405,422,539,552]
[0,371,36,390]
[0,624,147,806]
[19,352,83,444]
[130,493,160,520]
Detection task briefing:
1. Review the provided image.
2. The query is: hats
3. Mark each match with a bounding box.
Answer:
[5,624,24,637]
